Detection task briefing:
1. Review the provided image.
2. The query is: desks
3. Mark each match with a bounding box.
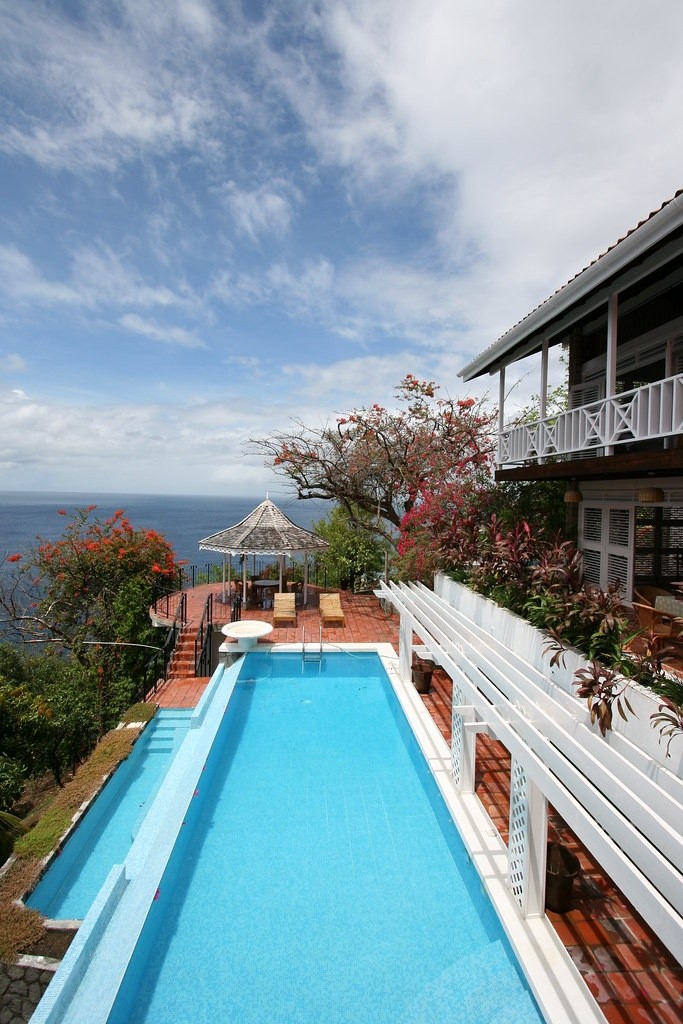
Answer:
[654,595,683,620]
[252,580,279,609]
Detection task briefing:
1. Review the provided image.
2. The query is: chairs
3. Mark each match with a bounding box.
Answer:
[234,573,304,609]
[631,585,683,661]
[319,593,345,627]
[273,592,297,627]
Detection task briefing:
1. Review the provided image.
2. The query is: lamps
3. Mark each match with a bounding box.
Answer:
[564,478,583,502]
[638,471,664,502]
